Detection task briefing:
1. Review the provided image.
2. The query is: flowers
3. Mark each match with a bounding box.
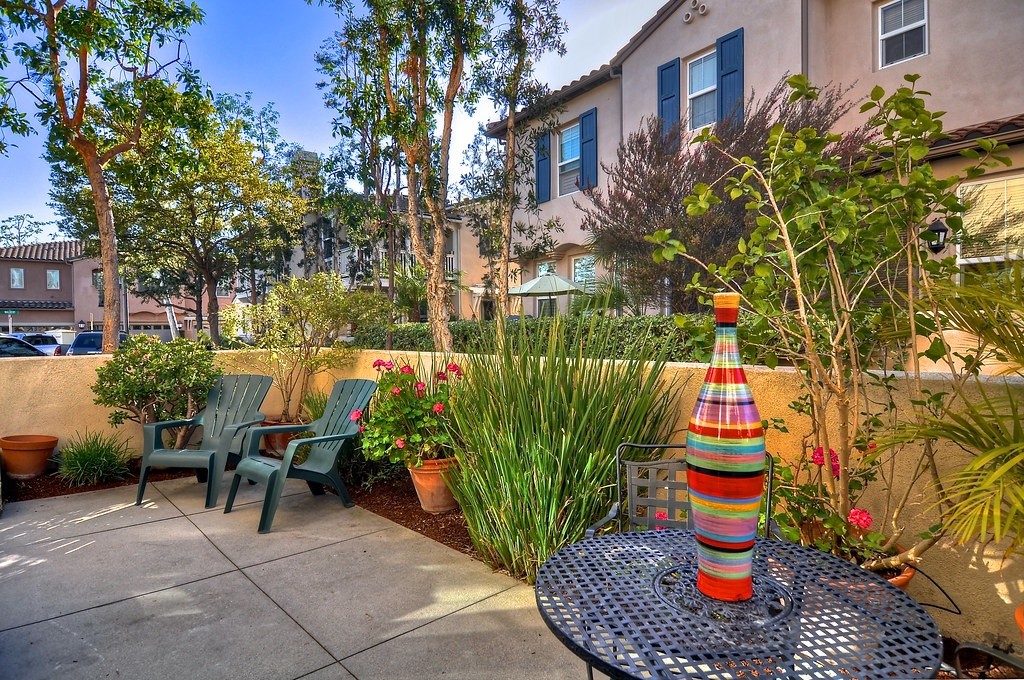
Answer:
[354,356,473,469]
[651,98,997,581]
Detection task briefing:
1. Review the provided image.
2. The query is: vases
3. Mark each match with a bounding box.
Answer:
[820,529,916,609]
[408,453,461,514]
[0,433,59,481]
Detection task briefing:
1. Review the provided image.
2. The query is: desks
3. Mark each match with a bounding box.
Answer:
[536,511,945,680]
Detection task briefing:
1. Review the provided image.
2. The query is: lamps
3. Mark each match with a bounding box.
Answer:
[927,216,950,253]
[78,320,86,329]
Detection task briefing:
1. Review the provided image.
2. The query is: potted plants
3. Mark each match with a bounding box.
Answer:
[222,271,403,457]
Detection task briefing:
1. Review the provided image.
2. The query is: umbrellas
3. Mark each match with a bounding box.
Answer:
[509,269,594,316]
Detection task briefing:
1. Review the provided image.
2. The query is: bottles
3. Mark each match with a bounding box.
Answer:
[685,293,766,602]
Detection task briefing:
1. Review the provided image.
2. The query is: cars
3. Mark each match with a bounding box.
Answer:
[0,330,79,357]
[65,329,136,356]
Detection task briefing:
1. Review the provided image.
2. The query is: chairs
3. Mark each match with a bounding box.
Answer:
[136,375,274,508]
[578,441,774,680]
[225,380,378,534]
